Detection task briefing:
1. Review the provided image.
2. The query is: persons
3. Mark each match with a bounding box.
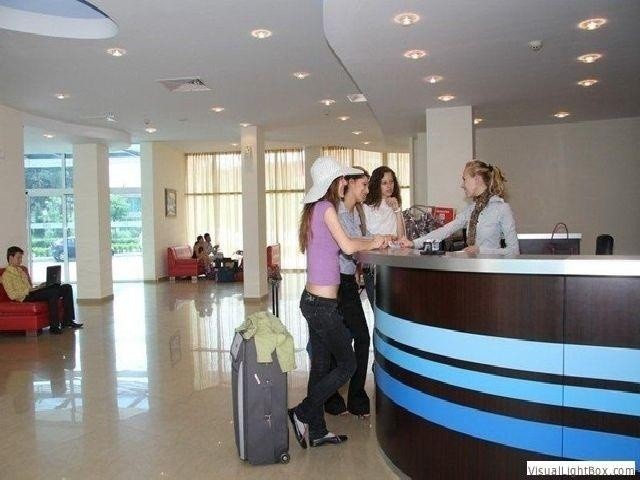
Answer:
[1,247,83,334]
[324,166,395,417]
[192,235,215,280]
[287,155,393,450]
[396,160,522,257]
[361,166,409,311]
[201,233,220,257]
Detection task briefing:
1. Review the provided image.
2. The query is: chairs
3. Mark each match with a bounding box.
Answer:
[1,264,67,337]
[595,232,616,255]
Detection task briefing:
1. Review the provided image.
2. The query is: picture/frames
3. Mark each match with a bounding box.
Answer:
[165,187,178,218]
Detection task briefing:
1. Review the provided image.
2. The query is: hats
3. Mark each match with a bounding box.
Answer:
[304,156,364,203]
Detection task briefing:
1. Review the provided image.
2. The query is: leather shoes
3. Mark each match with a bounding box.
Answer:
[310,435,347,447]
[50,327,62,334]
[288,407,307,449]
[61,320,83,327]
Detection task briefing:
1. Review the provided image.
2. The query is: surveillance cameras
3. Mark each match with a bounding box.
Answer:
[25,190,28,194]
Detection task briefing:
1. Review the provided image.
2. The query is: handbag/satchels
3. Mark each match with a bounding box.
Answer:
[217,271,234,282]
[192,244,203,258]
[403,206,436,241]
[544,223,578,255]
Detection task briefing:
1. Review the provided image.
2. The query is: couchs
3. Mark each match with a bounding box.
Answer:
[166,245,205,282]
[267,243,282,279]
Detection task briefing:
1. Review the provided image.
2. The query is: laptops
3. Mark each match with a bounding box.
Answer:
[28,265,61,293]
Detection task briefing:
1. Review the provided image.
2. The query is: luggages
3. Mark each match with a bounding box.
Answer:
[230,277,290,465]
[306,283,365,372]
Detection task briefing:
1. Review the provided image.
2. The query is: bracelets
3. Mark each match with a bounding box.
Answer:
[395,207,402,214]
